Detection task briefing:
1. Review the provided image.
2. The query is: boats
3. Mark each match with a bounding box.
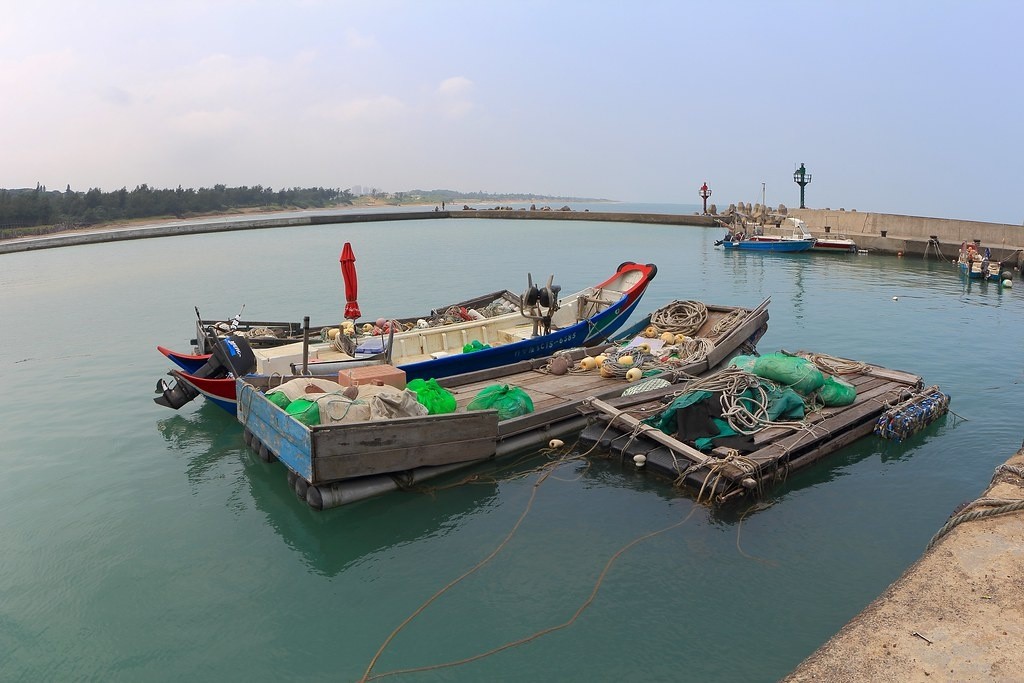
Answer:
[232,296,771,510]
[150,241,660,417]
[958,240,1004,281]
[713,212,856,254]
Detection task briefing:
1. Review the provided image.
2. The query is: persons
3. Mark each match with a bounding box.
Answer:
[435,201,445,211]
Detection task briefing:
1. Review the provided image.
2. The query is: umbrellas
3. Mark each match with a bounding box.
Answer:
[340,242,361,323]
